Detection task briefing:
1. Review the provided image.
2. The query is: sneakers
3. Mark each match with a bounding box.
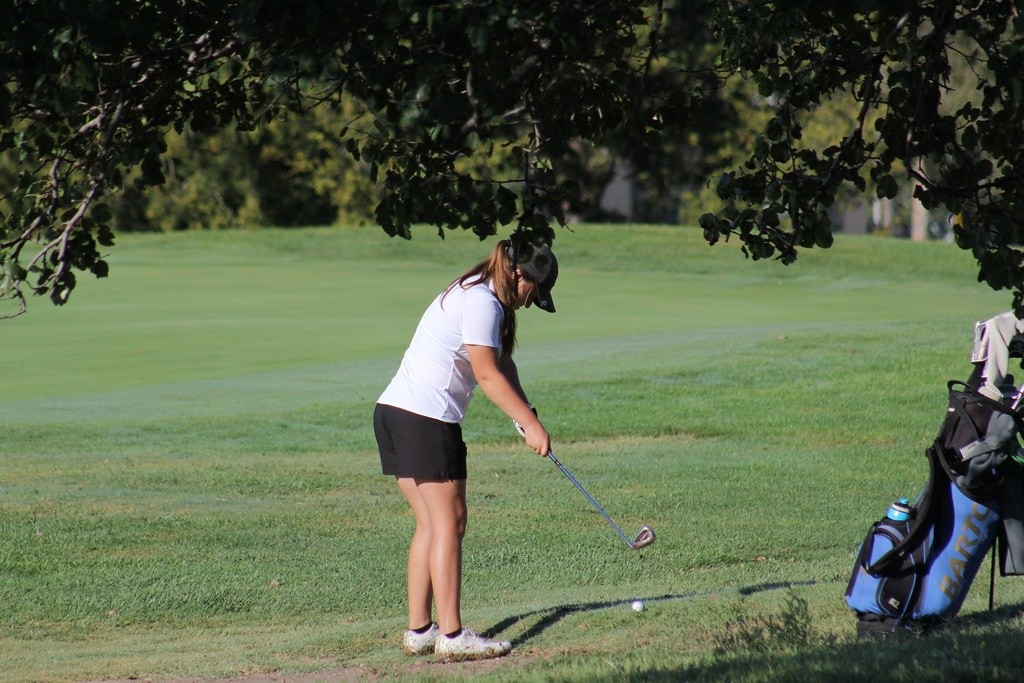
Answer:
[434,624,512,664]
[403,621,439,656]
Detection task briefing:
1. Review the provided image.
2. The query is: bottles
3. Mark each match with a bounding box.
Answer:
[886,498,912,522]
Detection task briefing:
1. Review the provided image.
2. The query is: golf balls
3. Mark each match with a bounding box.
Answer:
[632,601,644,612]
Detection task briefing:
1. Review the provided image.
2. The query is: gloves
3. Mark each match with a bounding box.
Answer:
[513,400,537,438]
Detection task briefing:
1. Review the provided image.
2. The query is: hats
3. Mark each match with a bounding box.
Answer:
[507,242,559,313]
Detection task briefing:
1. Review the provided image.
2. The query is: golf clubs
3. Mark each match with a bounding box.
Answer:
[546,450,657,551]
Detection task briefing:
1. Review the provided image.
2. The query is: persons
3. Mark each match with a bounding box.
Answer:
[374,233,558,662]
